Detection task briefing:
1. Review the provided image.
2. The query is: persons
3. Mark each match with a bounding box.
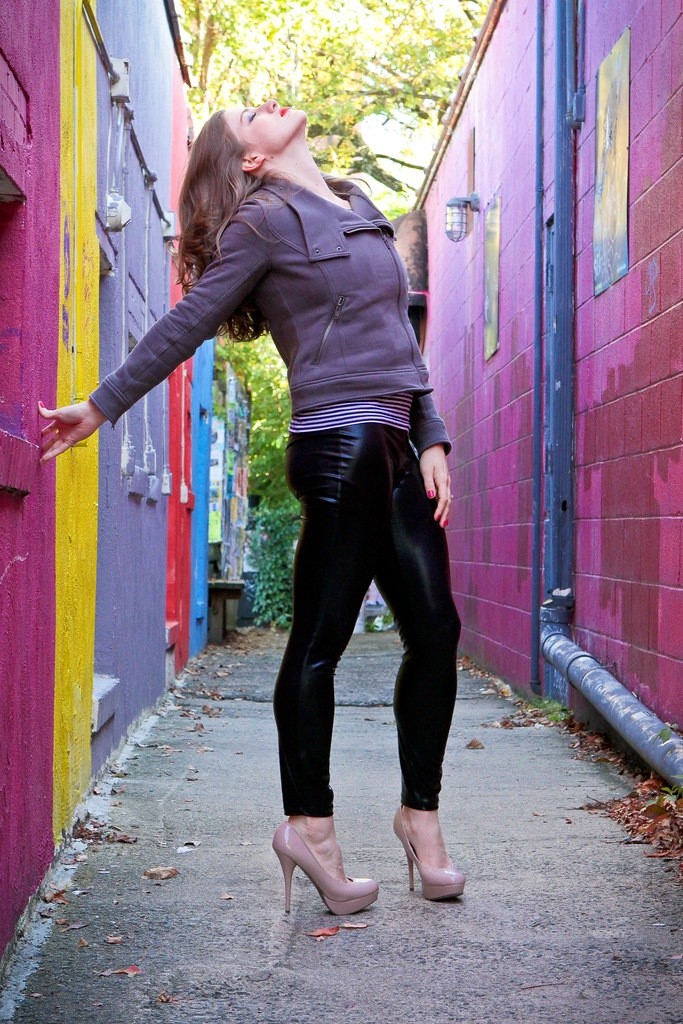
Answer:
[38,99,466,915]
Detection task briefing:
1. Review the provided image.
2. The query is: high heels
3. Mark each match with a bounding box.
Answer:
[394,807,465,899]
[273,822,380,914]
